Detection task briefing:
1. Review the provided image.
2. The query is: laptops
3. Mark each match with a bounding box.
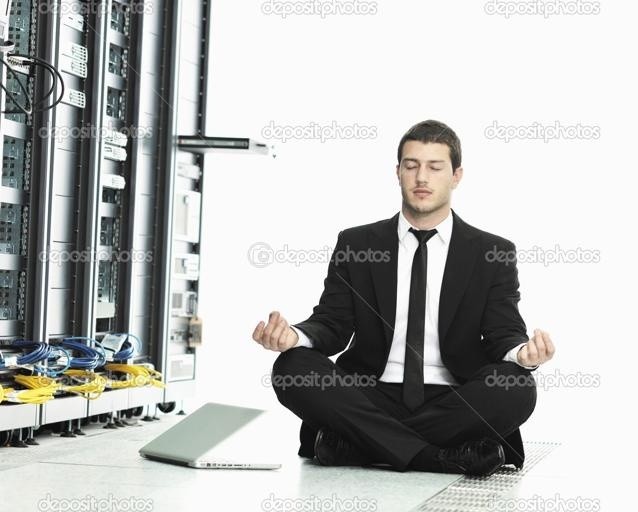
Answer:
[139,402,282,470]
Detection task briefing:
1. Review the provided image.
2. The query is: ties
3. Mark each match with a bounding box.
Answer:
[401,228,438,416]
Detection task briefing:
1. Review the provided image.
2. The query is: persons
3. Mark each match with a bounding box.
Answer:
[251,119,555,477]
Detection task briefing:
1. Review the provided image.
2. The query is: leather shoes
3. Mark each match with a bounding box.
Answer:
[442,439,505,477]
[313,426,366,465]
